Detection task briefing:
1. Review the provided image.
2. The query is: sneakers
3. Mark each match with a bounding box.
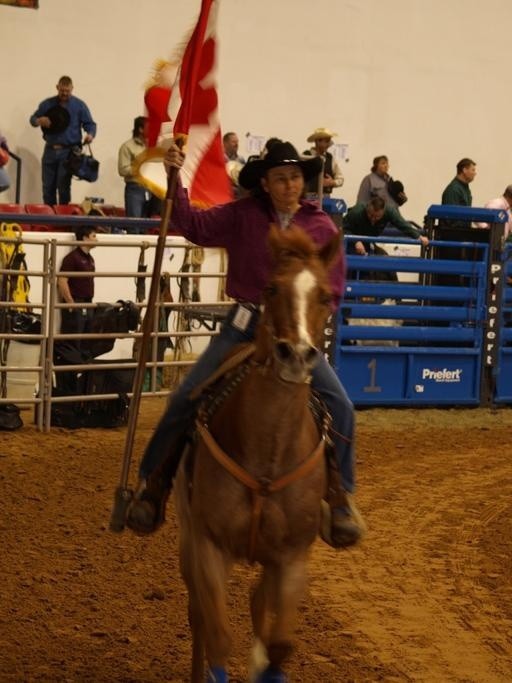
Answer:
[125,496,165,532]
[331,506,361,546]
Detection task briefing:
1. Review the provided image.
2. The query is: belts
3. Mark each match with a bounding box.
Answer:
[46,143,68,150]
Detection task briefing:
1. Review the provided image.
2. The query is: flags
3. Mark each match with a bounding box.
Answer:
[130,0,234,211]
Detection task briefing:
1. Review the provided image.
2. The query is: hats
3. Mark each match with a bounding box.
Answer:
[388,177,405,205]
[42,106,71,135]
[239,142,323,192]
[307,127,337,142]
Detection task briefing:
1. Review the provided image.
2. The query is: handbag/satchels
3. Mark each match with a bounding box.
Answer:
[69,143,99,182]
[37,340,129,428]
[11,313,41,343]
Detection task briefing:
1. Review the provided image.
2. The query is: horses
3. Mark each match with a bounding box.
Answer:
[169,221,346,683]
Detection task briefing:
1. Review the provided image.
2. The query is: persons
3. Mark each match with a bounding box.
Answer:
[224,132,245,199]
[30,75,97,208]
[117,116,162,218]
[475,185,511,285]
[435,160,475,284]
[341,195,430,346]
[124,137,365,548]
[301,127,345,200]
[356,157,406,211]
[0,135,11,194]
[55,225,97,374]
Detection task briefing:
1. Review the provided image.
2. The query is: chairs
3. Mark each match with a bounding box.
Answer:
[4,204,138,232]
